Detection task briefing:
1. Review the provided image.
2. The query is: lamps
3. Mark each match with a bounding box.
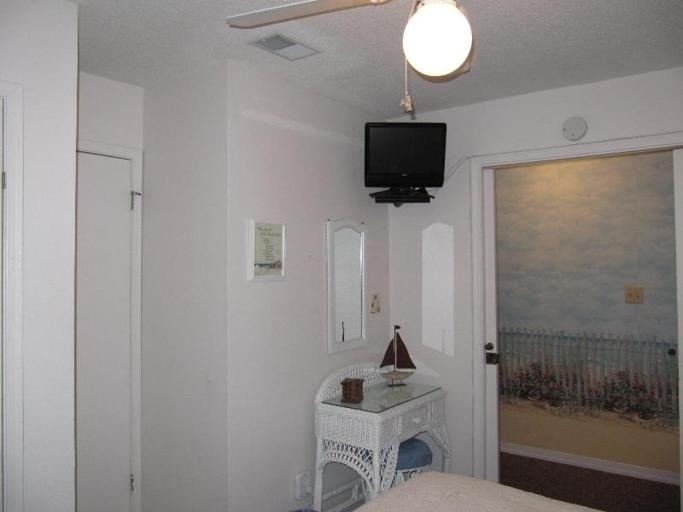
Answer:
[400,0,475,114]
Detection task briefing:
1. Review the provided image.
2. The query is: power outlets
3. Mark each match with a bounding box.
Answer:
[295,471,314,500]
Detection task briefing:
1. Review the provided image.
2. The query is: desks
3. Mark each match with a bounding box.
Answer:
[308,364,452,512]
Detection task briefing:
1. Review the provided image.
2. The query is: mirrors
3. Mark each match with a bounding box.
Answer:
[323,218,368,353]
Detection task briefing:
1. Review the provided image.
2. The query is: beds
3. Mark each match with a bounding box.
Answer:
[351,469,605,512]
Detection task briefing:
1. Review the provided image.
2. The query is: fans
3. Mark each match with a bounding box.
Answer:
[226,0,392,28]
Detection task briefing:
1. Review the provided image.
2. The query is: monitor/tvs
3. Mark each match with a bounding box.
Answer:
[364,121,447,188]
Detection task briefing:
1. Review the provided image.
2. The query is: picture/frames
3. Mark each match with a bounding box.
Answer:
[248,216,286,281]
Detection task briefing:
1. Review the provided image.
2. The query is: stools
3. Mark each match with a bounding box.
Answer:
[356,436,432,503]
